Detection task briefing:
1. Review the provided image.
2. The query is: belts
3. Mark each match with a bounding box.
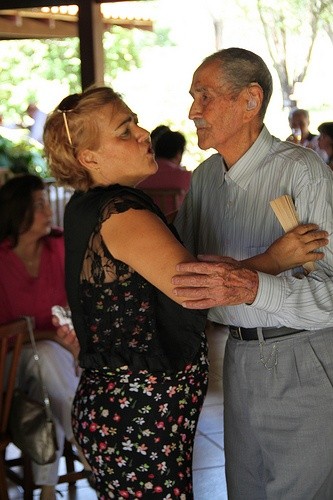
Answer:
[229,326,307,341]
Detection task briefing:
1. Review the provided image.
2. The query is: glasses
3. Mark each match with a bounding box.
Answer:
[58,93,81,146]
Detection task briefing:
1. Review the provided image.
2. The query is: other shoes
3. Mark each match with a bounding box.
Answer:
[82,469,100,491]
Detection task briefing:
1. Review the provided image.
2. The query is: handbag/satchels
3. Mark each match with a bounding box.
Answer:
[7,395,56,465]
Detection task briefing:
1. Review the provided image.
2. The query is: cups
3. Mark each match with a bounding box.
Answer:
[294,128,302,145]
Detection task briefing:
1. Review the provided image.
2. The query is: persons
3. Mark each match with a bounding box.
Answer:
[284,110,333,171]
[43,88,329,500]
[135,126,193,221]
[0,174,90,499]
[0,103,47,145]
[49,47,333,500]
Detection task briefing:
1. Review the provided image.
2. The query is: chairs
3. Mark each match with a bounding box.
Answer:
[0,317,93,500]
[42,178,72,226]
[139,188,185,223]
